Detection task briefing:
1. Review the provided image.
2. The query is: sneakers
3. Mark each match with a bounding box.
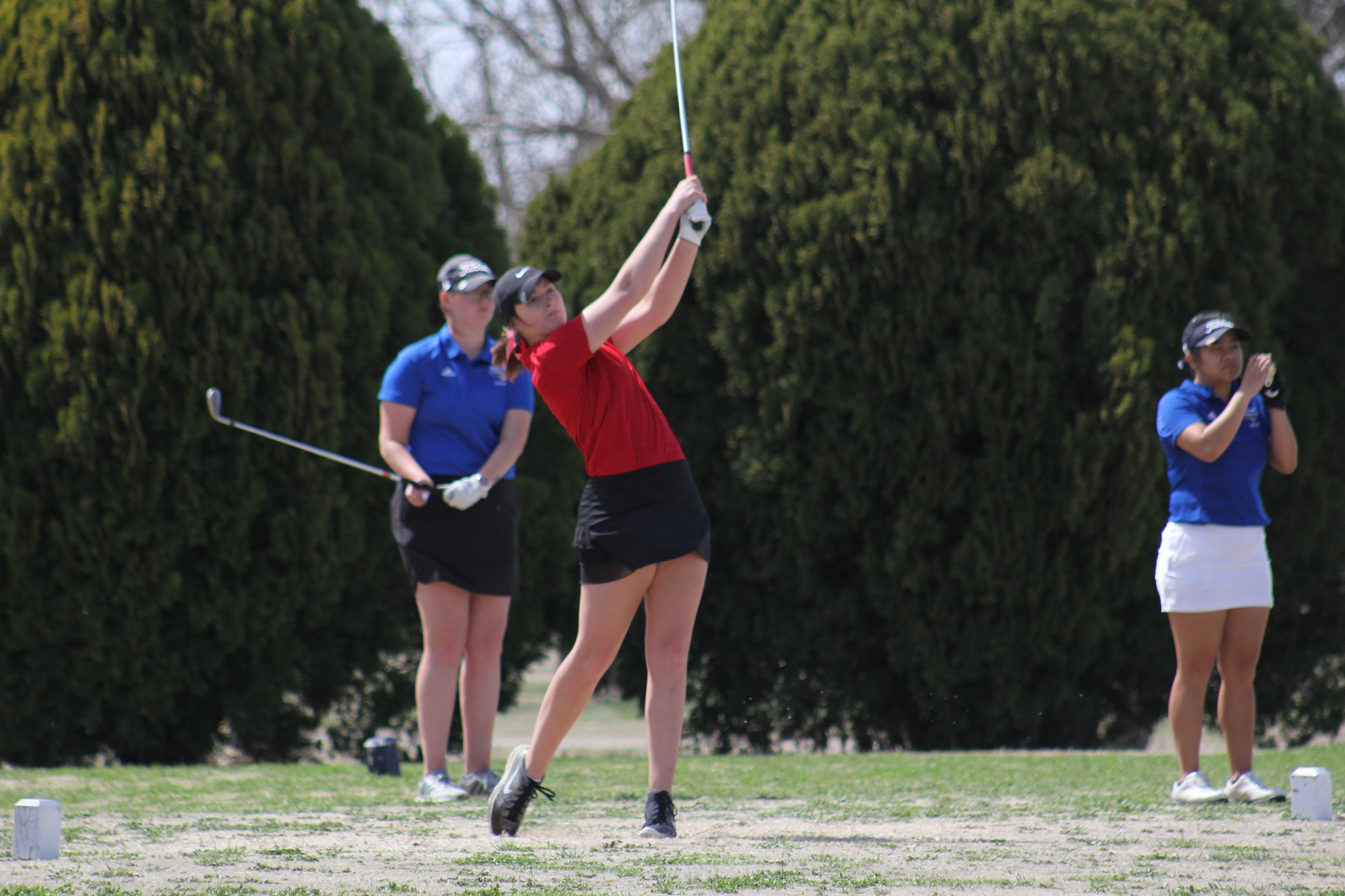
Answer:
[639,790,677,838]
[414,768,468,802]
[461,767,504,796]
[1225,771,1286,805]
[1172,771,1228,804]
[487,743,556,836]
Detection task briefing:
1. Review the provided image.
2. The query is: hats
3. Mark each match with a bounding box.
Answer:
[493,265,562,324]
[436,253,497,293]
[1182,313,1252,355]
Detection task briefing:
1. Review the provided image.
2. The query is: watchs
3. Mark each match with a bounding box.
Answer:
[476,474,490,491]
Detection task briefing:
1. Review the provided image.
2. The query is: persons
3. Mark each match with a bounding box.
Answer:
[379,255,536,802]
[1155,311,1298,809]
[488,177,713,842]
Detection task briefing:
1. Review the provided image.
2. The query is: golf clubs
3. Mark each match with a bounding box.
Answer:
[670,0,702,230]
[206,387,443,496]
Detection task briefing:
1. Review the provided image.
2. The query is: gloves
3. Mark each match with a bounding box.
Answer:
[677,198,712,247]
[442,473,491,511]
[1259,363,1287,412]
